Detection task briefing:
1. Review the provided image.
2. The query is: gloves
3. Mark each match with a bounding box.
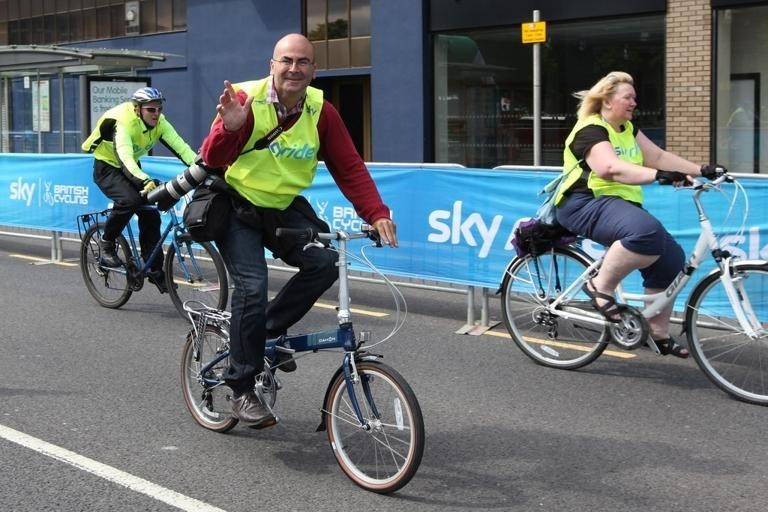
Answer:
[141,178,161,192]
[657,170,693,186]
[700,162,726,180]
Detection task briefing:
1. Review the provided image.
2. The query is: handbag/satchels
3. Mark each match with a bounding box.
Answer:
[536,173,567,226]
[183,175,231,242]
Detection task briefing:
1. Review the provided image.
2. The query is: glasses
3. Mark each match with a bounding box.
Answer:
[140,106,162,114]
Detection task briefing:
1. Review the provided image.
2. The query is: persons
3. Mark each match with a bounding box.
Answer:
[555,71,727,359]
[200,33,398,430]
[81,87,198,293]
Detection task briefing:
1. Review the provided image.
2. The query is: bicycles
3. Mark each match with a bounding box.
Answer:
[76,183,228,324]
[495,168,768,404]
[180,224,425,494]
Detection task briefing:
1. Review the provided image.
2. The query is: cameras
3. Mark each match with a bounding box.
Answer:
[145,161,224,212]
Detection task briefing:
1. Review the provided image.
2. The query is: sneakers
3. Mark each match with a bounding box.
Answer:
[265,348,297,373]
[231,391,273,426]
[148,270,178,293]
[99,233,121,267]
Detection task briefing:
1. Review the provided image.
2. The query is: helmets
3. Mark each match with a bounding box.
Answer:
[131,86,163,106]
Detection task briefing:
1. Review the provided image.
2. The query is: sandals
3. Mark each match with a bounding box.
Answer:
[581,280,621,323]
[644,334,689,359]
